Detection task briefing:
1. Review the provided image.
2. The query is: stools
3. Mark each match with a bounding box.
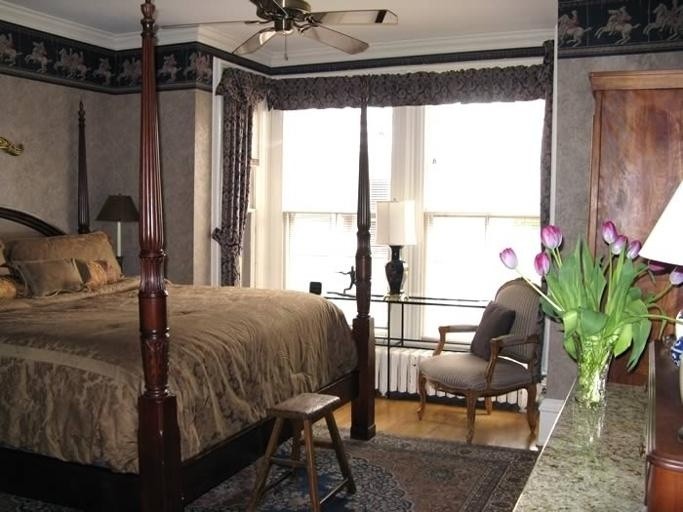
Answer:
[244,392,356,512]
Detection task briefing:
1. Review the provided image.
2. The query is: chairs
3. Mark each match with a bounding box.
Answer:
[414,276,546,444]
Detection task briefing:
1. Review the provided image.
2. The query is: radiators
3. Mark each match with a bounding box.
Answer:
[375,343,528,413]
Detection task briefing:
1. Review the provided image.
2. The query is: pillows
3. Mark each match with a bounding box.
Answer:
[470,300,516,361]
[0,230,129,299]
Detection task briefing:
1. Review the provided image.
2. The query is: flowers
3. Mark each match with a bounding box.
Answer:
[497,217,683,404]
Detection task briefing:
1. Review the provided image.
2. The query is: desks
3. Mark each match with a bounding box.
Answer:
[511,374,646,512]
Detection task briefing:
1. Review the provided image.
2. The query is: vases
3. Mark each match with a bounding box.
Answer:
[573,329,621,410]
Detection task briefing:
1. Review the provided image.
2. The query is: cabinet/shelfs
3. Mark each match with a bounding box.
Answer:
[322,295,493,401]
[639,340,683,512]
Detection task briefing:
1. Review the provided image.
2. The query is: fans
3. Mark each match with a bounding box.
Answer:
[228,0,398,63]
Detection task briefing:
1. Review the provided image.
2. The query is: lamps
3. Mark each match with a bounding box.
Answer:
[637,181,683,366]
[375,197,417,301]
[94,192,138,273]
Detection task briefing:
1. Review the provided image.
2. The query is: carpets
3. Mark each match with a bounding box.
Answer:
[7,419,537,508]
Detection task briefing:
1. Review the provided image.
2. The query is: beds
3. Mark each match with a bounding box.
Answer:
[0,0,375,512]
